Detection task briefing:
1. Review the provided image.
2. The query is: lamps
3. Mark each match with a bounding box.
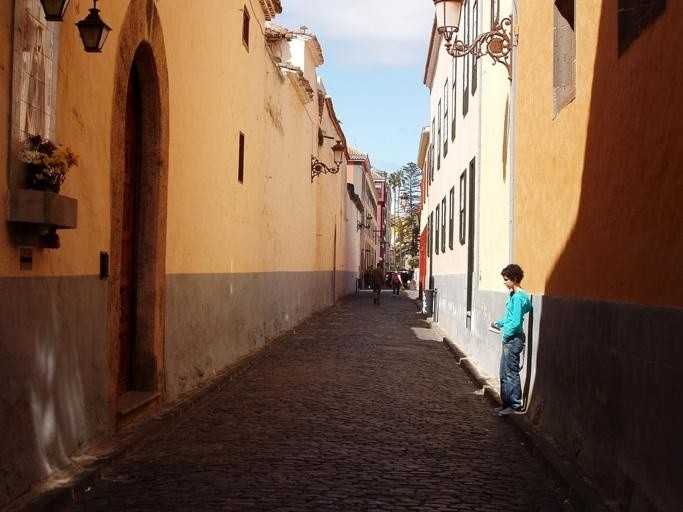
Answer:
[40,0,111,52]
[434,0,512,82]
[311,140,344,184]
[399,193,421,217]
[358,214,373,232]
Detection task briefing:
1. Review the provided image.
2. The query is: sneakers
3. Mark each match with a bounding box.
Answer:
[494,407,518,416]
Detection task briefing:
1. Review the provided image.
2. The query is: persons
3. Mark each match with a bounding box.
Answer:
[370,263,384,305]
[390,270,403,297]
[491,264,533,416]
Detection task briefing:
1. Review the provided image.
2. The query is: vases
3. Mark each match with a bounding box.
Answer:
[29,164,62,193]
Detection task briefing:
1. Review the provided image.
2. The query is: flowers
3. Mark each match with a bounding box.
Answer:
[18,137,80,186]
